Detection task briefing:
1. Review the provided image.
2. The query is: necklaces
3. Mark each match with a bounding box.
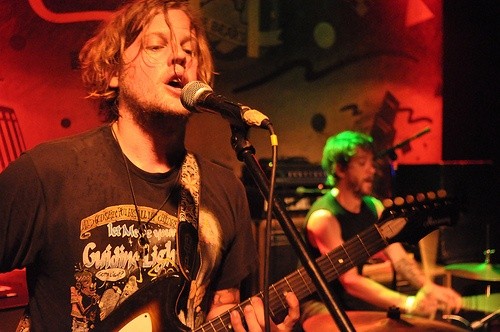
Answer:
[111,120,180,248]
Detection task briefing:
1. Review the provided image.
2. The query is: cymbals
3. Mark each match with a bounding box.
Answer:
[302,311,463,332]
[444,262,500,281]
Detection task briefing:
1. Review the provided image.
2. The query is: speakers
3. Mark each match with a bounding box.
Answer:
[270,234,304,278]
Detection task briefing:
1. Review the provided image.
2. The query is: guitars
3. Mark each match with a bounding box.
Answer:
[92,188,458,332]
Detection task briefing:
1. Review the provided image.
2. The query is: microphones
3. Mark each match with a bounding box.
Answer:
[180,80,272,129]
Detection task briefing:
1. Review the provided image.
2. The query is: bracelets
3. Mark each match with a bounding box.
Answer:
[406,295,414,309]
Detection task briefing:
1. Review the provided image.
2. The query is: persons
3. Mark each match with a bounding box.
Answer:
[419,228,473,281]
[0,0,300,332]
[305,132,462,320]
